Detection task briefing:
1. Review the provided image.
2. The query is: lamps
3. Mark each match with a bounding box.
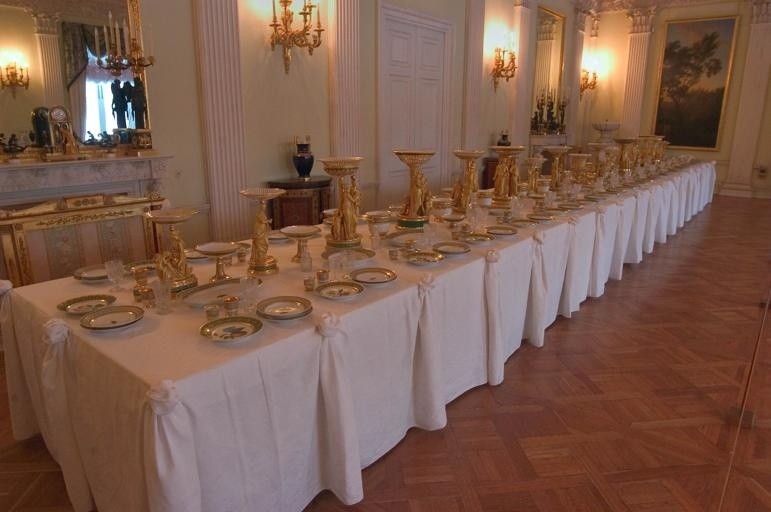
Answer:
[580,52,612,103]
[269,0,325,75]
[488,17,519,92]
[0,48,31,100]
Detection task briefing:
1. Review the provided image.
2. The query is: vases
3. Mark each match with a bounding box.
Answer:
[293,143,314,178]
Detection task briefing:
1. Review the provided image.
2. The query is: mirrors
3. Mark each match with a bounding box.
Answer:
[0,0,161,165]
[531,6,564,121]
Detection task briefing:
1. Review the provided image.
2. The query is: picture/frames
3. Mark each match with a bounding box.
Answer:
[650,15,740,152]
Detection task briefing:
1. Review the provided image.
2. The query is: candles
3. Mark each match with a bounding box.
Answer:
[94,21,131,76]
[108,10,155,73]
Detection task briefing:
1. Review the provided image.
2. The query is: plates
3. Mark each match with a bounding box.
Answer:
[528,213,553,222]
[318,281,365,301]
[197,318,265,347]
[72,239,291,285]
[57,293,118,316]
[320,250,375,262]
[408,251,443,265]
[577,177,652,212]
[386,230,428,246]
[463,233,495,244]
[177,277,263,312]
[546,204,584,215]
[484,225,518,236]
[511,219,538,228]
[350,267,397,285]
[433,240,471,255]
[79,305,144,338]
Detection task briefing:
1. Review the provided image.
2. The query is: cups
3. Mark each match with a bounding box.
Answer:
[431,177,550,239]
[204,304,220,320]
[302,276,315,292]
[256,296,313,320]
[504,211,512,222]
[462,223,472,233]
[151,280,172,315]
[389,249,399,262]
[239,270,258,315]
[317,270,331,281]
[449,223,459,238]
[223,294,240,317]
[497,217,504,224]
[405,240,418,251]
[361,207,438,263]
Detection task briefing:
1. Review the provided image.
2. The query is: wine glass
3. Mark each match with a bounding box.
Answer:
[104,260,125,291]
